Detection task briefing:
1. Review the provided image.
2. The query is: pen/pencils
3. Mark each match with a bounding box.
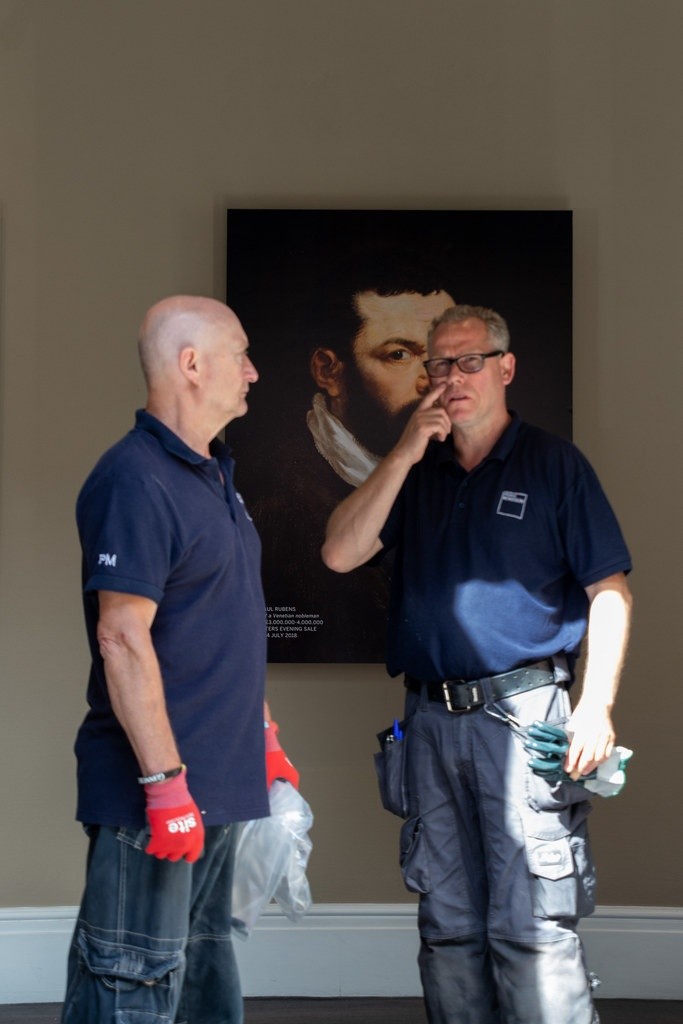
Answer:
[387,735,394,744]
[394,720,399,741]
[399,731,402,742]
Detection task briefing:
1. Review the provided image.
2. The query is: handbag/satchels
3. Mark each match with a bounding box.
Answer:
[372,704,413,819]
[482,685,597,811]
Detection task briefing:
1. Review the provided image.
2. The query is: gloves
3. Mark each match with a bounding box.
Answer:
[146,774,204,864]
[524,721,634,797]
[265,722,300,792]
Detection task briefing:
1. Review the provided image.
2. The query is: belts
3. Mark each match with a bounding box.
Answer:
[406,662,553,713]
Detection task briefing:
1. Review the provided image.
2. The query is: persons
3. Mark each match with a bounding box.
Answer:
[247,246,463,662]
[319,304,633,1024]
[61,295,298,1024]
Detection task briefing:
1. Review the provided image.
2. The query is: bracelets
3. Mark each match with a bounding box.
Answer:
[138,763,187,784]
[264,720,279,734]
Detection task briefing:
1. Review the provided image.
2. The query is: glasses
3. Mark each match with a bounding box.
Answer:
[423,350,503,378]
